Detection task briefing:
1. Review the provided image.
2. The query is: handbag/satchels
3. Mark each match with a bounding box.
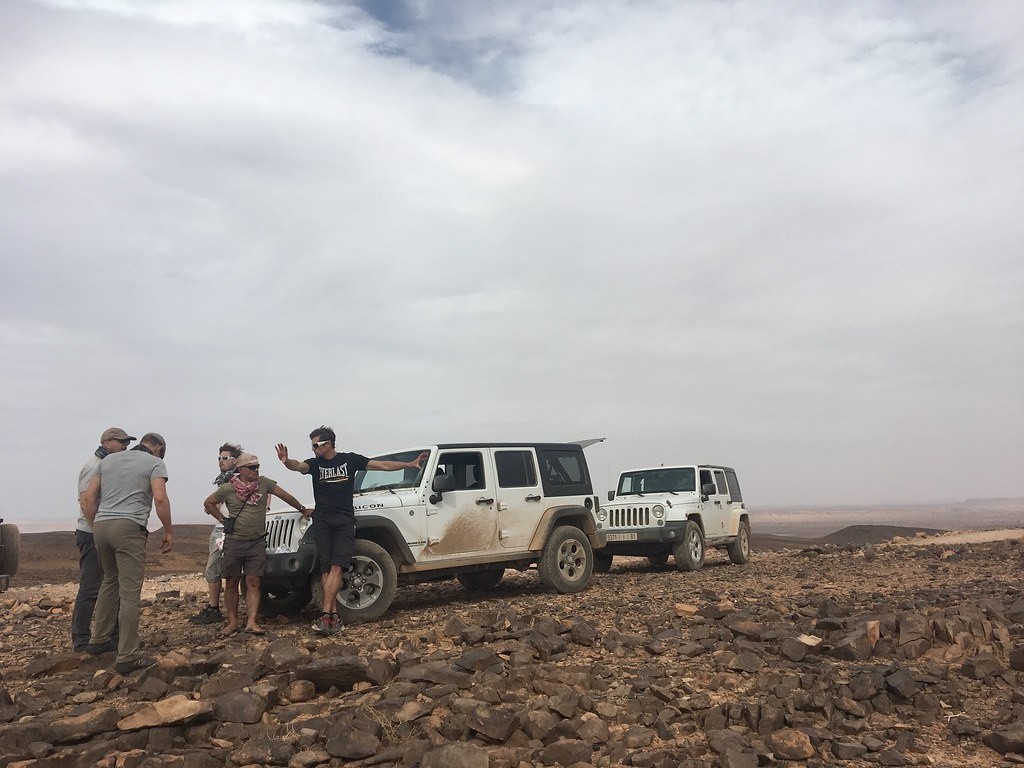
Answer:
[223,518,235,534]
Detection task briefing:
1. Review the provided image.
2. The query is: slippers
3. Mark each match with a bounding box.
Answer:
[244,624,266,634]
[221,625,239,636]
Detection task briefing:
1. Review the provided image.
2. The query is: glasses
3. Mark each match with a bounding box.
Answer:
[218,456,235,461]
[242,464,260,470]
[312,441,328,448]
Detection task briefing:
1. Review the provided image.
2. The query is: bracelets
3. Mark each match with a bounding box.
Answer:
[221,517,226,524]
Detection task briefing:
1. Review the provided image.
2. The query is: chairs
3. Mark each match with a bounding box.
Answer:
[414,468,444,492]
[647,479,663,490]
[467,465,483,488]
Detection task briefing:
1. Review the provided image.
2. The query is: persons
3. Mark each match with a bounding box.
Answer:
[432,466,483,492]
[275,424,429,634]
[72,428,314,674]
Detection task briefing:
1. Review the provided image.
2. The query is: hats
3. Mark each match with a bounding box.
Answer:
[145,433,166,459]
[101,428,137,443]
[231,453,258,474]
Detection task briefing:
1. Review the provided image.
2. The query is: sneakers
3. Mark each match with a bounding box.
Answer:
[194,611,222,625]
[189,608,208,621]
[311,613,330,636]
[87,640,117,655]
[116,656,160,677]
[330,614,342,634]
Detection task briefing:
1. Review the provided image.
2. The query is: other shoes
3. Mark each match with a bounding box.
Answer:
[73,643,88,654]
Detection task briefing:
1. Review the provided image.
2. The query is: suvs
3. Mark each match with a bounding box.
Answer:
[240,437,605,625]
[588,464,752,574]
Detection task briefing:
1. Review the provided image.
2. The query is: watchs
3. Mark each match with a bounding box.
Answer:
[300,506,306,513]
[265,505,271,510]
